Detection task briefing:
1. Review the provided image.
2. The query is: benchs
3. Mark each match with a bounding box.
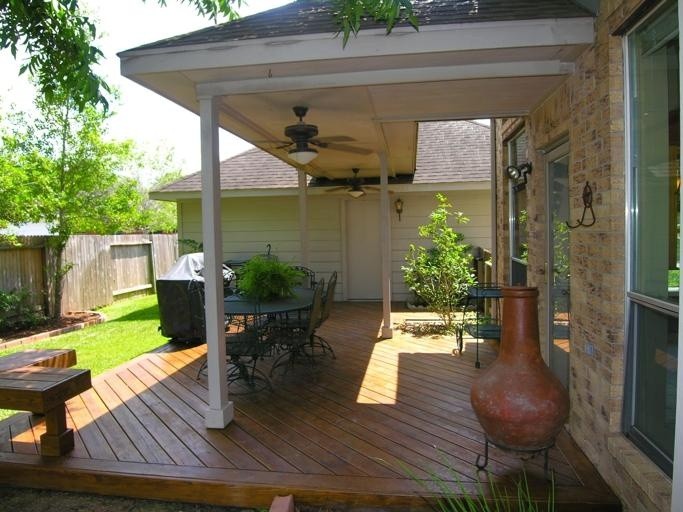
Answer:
[0,347,93,459]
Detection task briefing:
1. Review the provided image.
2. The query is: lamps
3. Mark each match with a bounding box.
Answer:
[506,161,532,193]
[394,198,403,221]
[287,142,319,165]
[347,186,364,198]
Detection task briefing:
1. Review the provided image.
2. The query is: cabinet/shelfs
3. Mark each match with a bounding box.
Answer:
[452,280,502,370]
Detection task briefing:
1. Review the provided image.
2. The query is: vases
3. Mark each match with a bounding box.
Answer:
[469,283,572,456]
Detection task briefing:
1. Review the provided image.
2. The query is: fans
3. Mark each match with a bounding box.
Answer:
[253,104,375,157]
[323,167,394,196]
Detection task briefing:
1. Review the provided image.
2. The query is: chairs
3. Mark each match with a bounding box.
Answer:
[185,264,338,398]
